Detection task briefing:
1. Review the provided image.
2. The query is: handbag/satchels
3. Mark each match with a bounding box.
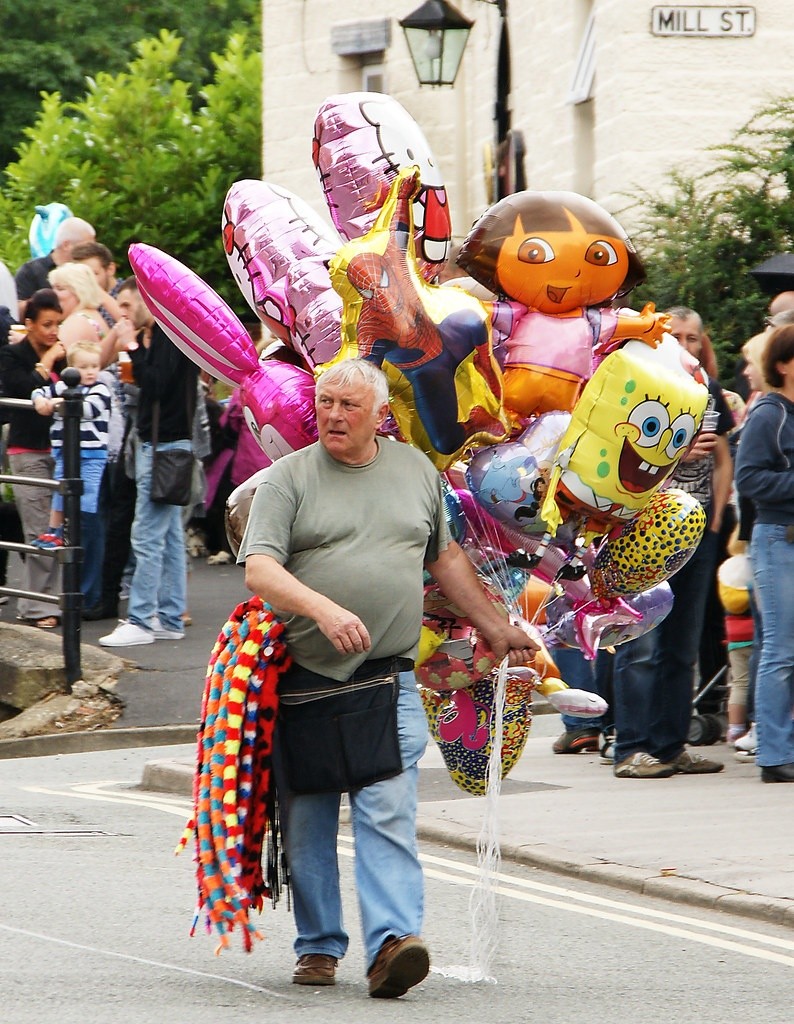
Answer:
[271,672,401,799]
[98,462,139,533]
[148,449,194,506]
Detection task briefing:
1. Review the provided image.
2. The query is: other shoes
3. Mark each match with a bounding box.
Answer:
[36,616,57,628]
[734,747,756,763]
[598,722,617,760]
[0,592,9,605]
[727,730,747,748]
[734,722,757,751]
[180,613,191,626]
[552,725,601,754]
[761,762,794,783]
[208,550,232,566]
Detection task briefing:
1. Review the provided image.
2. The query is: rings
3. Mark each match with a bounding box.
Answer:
[701,450,706,456]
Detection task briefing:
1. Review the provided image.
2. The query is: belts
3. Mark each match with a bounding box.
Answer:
[281,655,416,685]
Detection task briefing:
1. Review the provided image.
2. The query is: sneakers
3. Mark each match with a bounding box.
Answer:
[613,751,674,778]
[152,615,184,640]
[29,527,65,551]
[98,617,155,646]
[368,934,428,998]
[666,750,724,774]
[291,953,337,985]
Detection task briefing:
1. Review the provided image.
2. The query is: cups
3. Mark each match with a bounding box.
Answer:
[698,411,720,452]
[119,351,134,382]
[11,324,26,335]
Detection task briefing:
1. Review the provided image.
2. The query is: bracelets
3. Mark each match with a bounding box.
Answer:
[124,342,139,349]
[35,362,50,377]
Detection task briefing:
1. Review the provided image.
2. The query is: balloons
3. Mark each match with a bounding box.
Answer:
[415,473,608,795]
[127,91,451,390]
[460,184,710,659]
[238,168,513,469]
[28,202,73,259]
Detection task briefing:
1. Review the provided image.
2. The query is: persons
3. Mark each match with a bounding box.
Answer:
[552,651,598,755]
[611,304,735,779]
[71,241,124,327]
[697,290,794,783]
[31,339,112,551]
[0,289,63,629]
[98,277,197,647]
[80,368,135,620]
[183,369,230,565]
[8,262,111,349]
[15,217,96,321]
[596,648,617,764]
[237,358,541,998]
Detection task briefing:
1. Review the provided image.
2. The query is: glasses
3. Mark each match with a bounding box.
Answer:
[764,316,778,329]
